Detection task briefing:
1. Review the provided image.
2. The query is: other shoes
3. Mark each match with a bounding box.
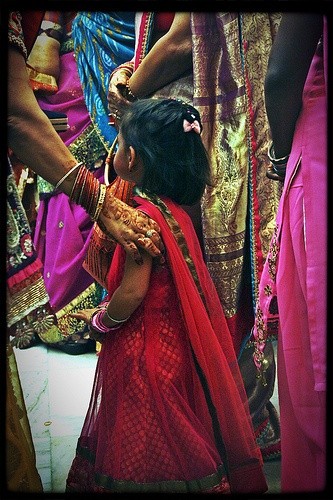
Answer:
[59,342,89,355]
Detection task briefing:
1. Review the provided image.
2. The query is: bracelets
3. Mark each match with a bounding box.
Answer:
[266,141,291,178]
[54,161,107,222]
[89,306,130,334]
[125,81,138,103]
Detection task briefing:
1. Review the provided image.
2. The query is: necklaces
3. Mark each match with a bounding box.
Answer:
[255,2,333,500]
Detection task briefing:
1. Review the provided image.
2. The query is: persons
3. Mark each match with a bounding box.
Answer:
[105,12,286,465]
[0,2,164,500]
[23,12,111,362]
[67,97,271,500]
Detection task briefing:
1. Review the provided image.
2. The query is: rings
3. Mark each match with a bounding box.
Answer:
[115,109,119,116]
[145,230,153,237]
